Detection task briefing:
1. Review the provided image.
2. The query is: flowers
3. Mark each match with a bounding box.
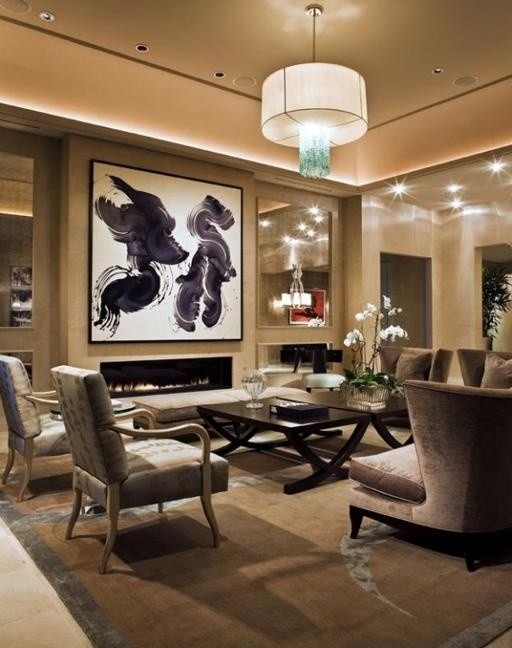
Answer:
[343,294,409,396]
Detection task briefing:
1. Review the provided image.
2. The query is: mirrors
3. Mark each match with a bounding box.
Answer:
[1,149,36,327]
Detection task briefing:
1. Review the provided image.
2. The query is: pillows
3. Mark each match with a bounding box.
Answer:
[394,346,431,385]
[478,352,511,388]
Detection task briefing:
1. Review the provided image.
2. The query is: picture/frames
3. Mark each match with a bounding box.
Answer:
[287,288,326,325]
[257,196,332,329]
[86,158,245,342]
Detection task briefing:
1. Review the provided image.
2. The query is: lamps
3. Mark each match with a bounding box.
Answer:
[260,4,369,180]
[280,264,312,309]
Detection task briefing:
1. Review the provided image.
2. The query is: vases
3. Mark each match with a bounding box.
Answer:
[341,385,389,410]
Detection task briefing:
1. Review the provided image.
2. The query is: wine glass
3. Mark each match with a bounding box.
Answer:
[241,368,268,408]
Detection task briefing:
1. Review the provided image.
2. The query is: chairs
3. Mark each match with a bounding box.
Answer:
[1,353,72,502]
[51,364,228,570]
[290,346,347,391]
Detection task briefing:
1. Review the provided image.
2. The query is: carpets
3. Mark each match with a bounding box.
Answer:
[0,451,512,647]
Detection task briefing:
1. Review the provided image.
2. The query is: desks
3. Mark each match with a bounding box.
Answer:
[50,401,137,415]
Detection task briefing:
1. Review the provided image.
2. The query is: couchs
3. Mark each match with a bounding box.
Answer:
[132,387,283,436]
[342,379,511,573]
[456,348,511,388]
[373,345,453,427]
